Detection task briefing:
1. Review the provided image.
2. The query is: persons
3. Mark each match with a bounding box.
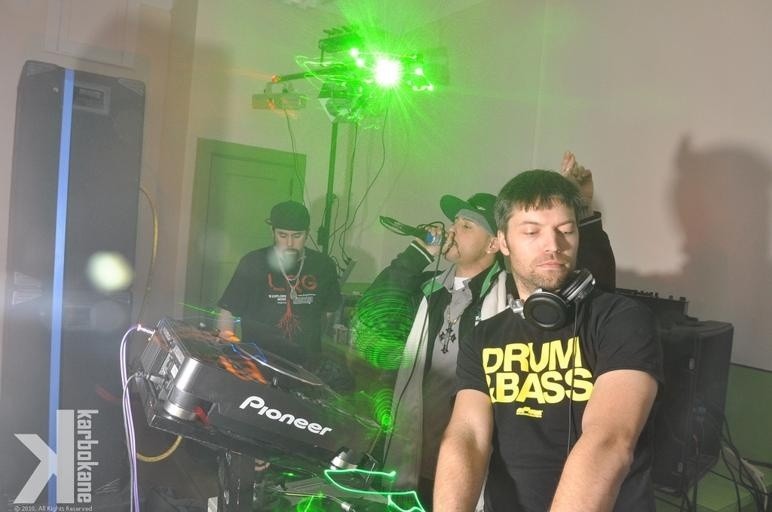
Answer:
[217,200,343,373]
[348,151,616,512]
[433,168,667,512]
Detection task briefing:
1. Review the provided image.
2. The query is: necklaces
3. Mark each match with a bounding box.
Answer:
[272,248,308,300]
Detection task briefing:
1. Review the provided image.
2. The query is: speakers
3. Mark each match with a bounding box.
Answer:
[655,317,734,498]
[7,58,147,364]
[615,288,690,321]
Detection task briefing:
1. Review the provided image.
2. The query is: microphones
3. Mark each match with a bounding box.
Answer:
[379,213,444,247]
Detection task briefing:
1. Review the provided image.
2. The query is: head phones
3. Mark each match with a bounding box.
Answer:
[507,259,595,331]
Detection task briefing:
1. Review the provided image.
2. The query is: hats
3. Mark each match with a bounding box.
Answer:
[271,200,309,230]
[440,193,498,237]
